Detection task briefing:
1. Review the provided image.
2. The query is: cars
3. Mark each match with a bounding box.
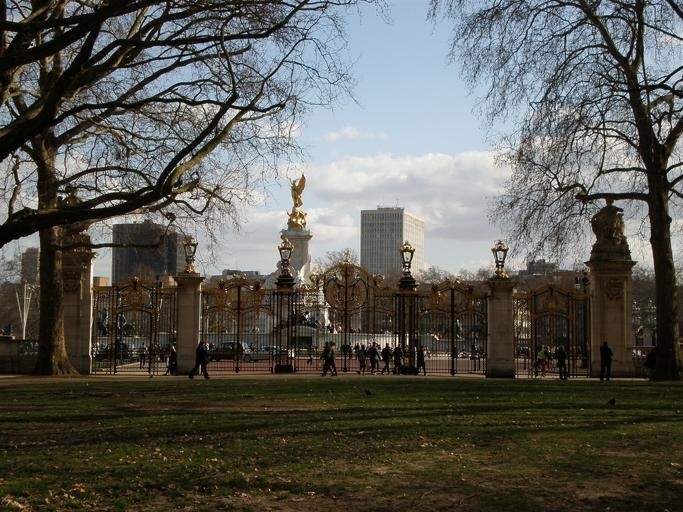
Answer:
[630,349,646,360]
[242,345,296,362]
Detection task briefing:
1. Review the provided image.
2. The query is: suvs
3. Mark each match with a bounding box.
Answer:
[94,341,140,361]
[206,340,250,361]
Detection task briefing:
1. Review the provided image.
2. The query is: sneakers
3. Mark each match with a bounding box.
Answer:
[600,380,604,382]
[189,376,193,379]
[424,373,426,375]
[564,378,567,381]
[204,376,210,379]
[415,373,419,375]
[606,379,610,382]
[321,371,400,376]
[560,378,563,380]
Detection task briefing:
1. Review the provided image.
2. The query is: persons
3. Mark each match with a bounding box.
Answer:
[139,339,426,381]
[288,178,303,208]
[557,347,568,381]
[599,341,614,381]
[535,346,554,377]
